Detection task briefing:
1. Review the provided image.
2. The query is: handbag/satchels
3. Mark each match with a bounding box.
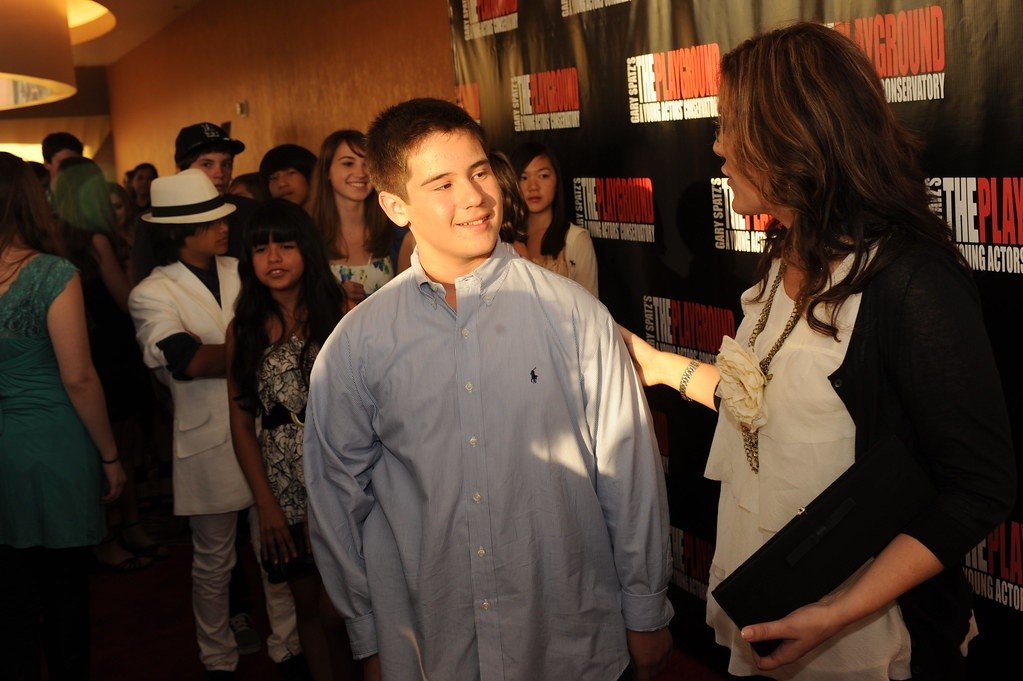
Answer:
[261,522,316,584]
[710,438,940,658]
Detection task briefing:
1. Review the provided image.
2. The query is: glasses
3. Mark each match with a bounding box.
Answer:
[711,118,737,143]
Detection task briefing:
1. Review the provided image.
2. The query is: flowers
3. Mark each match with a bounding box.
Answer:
[715,334,768,435]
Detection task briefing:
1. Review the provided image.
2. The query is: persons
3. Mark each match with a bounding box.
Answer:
[0,120,416,681]
[508,145,599,300]
[302,97,676,681]
[615,21,1023,681]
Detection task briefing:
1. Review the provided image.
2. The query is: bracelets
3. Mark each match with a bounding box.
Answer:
[680,359,701,402]
[103,457,118,464]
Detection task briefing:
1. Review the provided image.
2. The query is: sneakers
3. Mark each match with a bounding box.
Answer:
[228,613,261,655]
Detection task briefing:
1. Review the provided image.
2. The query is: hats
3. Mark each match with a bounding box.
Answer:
[174,122,245,169]
[139,168,237,224]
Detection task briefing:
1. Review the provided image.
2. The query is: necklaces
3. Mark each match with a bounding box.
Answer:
[733,258,804,474]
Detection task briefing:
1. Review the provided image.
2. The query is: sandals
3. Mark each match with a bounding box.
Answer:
[92,519,172,572]
